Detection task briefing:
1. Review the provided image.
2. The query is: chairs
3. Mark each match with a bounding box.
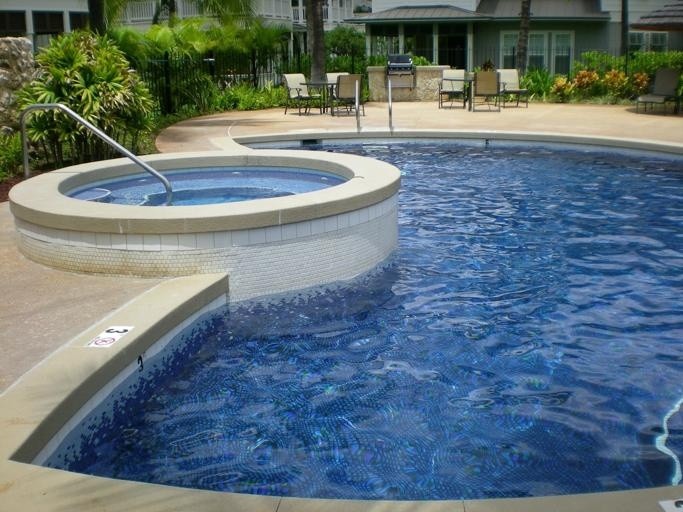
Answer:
[277,66,368,122]
[433,64,531,113]
[382,53,416,91]
[629,66,681,116]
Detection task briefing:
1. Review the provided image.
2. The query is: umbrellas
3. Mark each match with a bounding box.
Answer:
[629,0,682,31]
